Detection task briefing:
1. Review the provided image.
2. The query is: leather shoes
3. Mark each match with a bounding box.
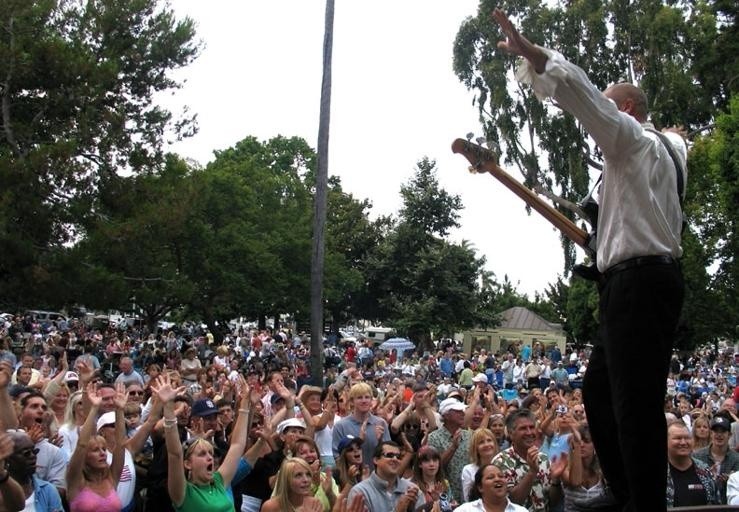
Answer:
[571,487,617,512]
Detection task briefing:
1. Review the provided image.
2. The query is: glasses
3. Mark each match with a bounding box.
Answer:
[381,451,402,460]
[406,424,418,429]
[130,391,144,396]
[489,413,502,418]
[16,448,40,457]
[346,446,361,452]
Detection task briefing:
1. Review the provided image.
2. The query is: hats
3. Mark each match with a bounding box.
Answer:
[667,387,677,397]
[439,397,469,416]
[191,397,219,417]
[458,353,465,358]
[65,370,80,382]
[96,411,116,434]
[558,361,563,365]
[473,351,479,355]
[271,394,286,404]
[550,380,555,385]
[447,391,464,402]
[709,416,733,430]
[295,384,322,403]
[276,417,306,436]
[690,407,702,418]
[337,434,364,455]
[471,373,488,384]
[520,388,528,395]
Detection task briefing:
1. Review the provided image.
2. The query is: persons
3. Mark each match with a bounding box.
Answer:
[1,311,738,511]
[491,7,688,510]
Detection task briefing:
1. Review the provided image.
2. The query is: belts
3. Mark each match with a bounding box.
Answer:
[597,253,673,293]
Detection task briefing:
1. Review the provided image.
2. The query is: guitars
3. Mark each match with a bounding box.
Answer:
[453,133,599,281]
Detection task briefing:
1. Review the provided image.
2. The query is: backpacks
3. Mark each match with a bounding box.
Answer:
[104,362,119,379]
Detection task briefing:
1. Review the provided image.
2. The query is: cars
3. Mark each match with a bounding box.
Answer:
[0,309,293,338]
[432,337,459,348]
[708,340,734,356]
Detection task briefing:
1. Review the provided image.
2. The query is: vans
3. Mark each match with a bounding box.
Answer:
[566,341,594,356]
[327,322,399,350]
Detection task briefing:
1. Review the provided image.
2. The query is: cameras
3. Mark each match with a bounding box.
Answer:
[556,405,567,413]
